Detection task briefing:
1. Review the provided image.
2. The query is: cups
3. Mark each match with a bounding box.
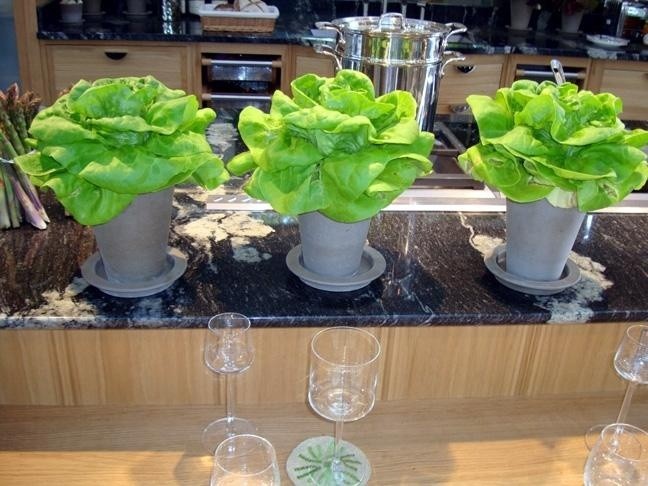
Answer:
[211,435,281,486]
[583,422,647,486]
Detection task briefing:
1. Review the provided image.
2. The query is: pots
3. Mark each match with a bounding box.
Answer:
[309,12,467,143]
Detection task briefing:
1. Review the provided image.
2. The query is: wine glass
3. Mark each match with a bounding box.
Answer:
[307,326,381,486]
[201,313,256,455]
[582,323,648,460]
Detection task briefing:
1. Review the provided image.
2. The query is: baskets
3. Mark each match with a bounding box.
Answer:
[199,2,280,33]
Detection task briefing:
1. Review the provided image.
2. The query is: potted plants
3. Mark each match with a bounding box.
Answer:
[454,80,648,296]
[227,72,436,294]
[15,70,234,299]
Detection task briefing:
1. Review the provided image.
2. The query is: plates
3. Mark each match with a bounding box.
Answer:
[586,33,627,50]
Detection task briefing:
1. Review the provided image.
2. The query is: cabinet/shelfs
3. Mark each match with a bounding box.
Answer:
[433,55,505,116]
[591,60,647,119]
[291,46,337,83]
[40,41,195,109]
